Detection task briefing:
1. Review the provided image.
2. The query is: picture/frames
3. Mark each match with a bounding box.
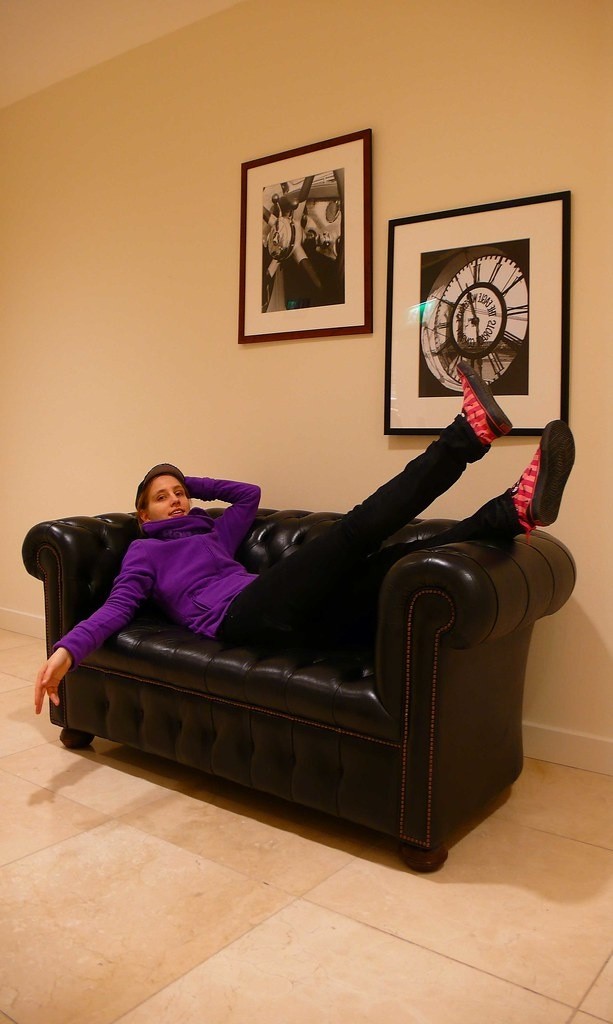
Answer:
[236,129,374,343]
[383,190,572,436]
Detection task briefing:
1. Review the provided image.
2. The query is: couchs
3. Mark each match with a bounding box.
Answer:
[21,507,577,872]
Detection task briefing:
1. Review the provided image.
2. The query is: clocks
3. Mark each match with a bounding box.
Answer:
[434,256,528,385]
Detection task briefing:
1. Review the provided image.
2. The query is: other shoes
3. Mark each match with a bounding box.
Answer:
[455,361,512,448]
[511,420,576,533]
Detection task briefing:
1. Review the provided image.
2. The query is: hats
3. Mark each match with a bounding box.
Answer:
[135,463,185,508]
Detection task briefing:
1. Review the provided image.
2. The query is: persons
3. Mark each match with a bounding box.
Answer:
[34,362,574,714]
